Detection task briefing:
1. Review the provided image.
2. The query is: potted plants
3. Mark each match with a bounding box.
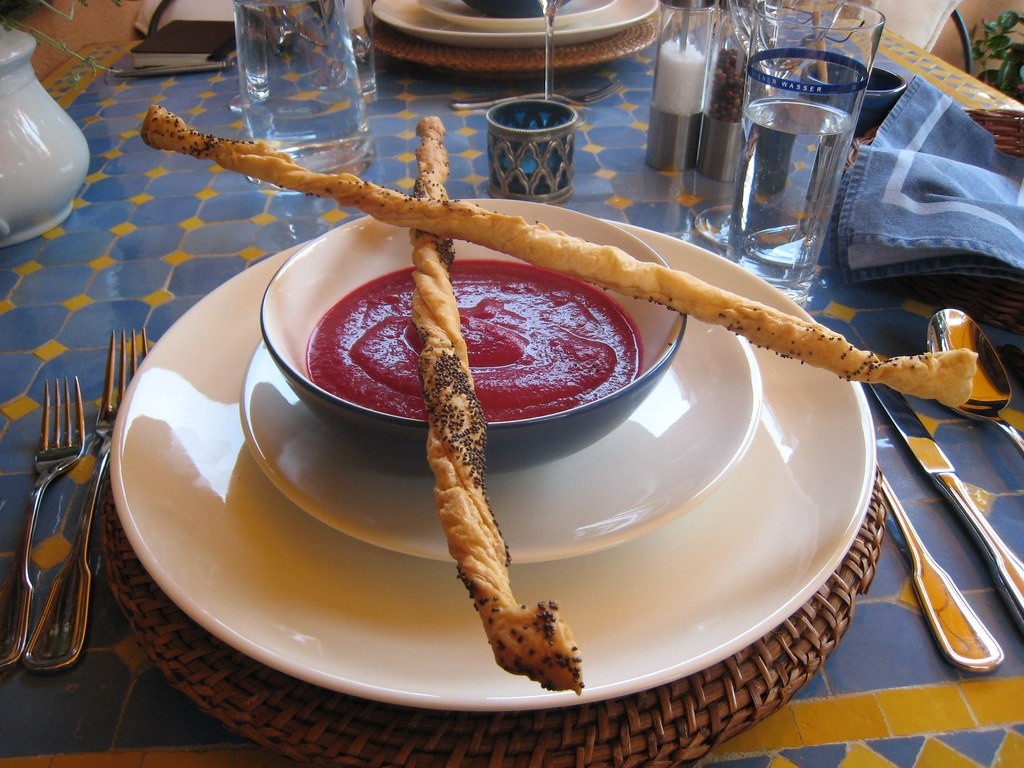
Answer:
[966,10,1024,105]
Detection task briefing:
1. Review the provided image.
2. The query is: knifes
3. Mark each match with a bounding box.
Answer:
[869,382,1024,628]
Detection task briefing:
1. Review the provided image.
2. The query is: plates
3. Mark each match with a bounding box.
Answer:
[372,0,660,48]
[110,218,876,711]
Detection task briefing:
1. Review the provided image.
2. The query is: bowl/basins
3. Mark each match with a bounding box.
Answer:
[799,60,908,138]
[462,0,570,18]
[260,198,687,431]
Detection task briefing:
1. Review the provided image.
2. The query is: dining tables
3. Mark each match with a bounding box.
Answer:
[0,37,1024,768]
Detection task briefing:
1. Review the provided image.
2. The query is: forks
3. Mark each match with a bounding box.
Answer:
[448,79,625,108]
[0,327,149,671]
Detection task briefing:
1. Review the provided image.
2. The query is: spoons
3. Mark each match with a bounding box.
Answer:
[925,309,1024,457]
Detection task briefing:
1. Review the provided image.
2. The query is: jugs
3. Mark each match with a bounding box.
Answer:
[0,19,89,248]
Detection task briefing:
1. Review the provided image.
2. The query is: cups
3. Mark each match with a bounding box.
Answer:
[231,0,376,194]
[646,0,749,182]
[725,0,886,307]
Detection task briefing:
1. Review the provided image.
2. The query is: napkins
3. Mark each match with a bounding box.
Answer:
[825,78,1024,287]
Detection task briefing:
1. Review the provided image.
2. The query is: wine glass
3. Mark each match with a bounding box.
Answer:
[536,0,587,127]
[693,0,848,249]
